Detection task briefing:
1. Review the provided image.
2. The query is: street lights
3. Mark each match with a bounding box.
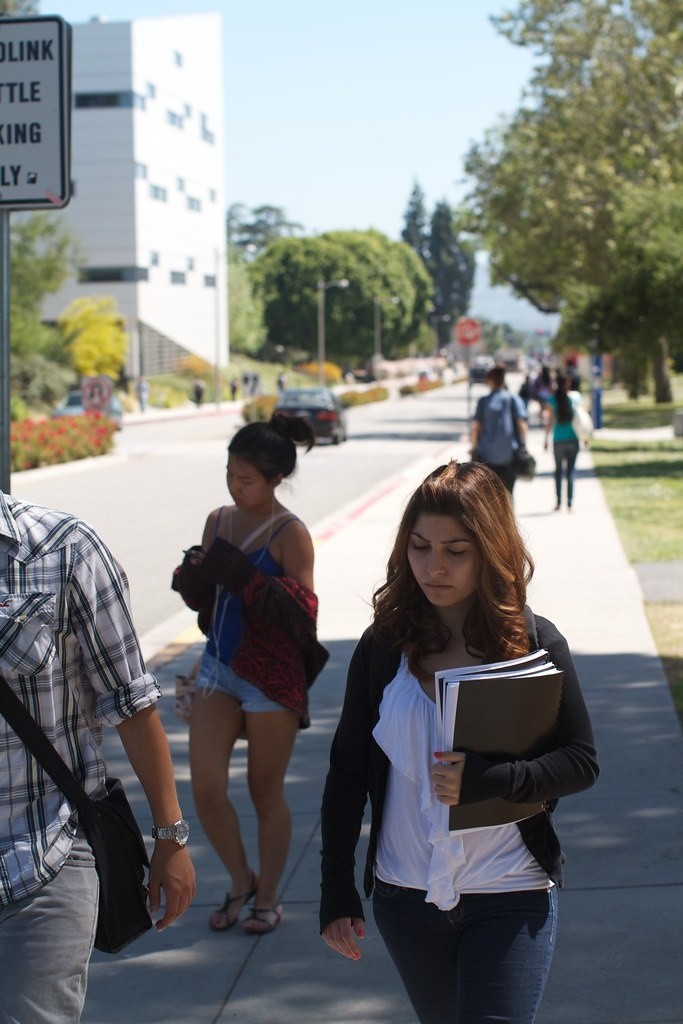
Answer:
[373,297,401,361]
[317,279,350,385]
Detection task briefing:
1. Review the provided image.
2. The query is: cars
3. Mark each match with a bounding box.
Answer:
[272,387,348,445]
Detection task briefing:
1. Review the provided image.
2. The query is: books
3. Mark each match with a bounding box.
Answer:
[434,648,564,838]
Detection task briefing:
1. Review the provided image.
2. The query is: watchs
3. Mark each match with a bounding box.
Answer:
[151,819,190,847]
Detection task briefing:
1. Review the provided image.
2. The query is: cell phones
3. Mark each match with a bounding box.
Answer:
[184,550,200,559]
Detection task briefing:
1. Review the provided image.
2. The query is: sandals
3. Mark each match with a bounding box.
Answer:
[208,869,258,931]
[241,901,282,934]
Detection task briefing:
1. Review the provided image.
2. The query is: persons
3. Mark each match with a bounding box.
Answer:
[543,375,588,514]
[241,374,259,398]
[276,372,287,392]
[89,387,102,400]
[519,359,584,412]
[192,378,205,407]
[172,412,318,935]
[0,490,197,1024]
[319,459,601,1024]
[469,367,528,496]
[230,378,238,401]
[136,375,150,412]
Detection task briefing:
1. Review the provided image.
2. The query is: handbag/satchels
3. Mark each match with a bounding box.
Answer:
[572,404,594,443]
[83,775,153,954]
[514,448,536,482]
[173,676,198,722]
